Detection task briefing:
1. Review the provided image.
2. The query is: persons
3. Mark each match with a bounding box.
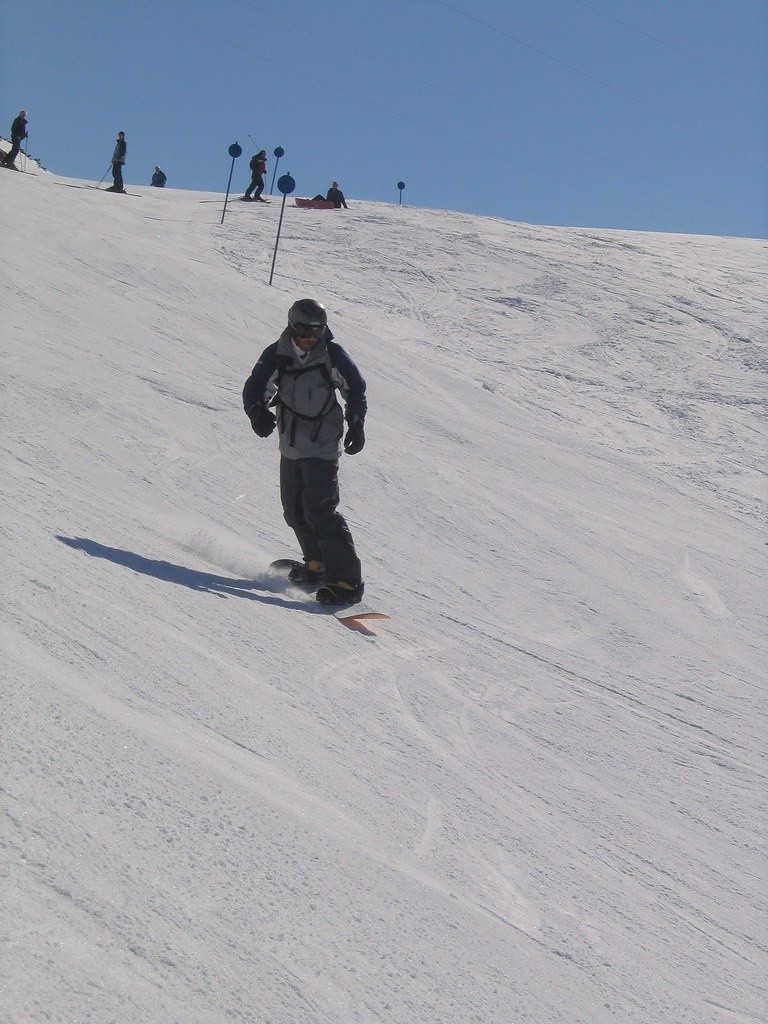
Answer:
[245,150,267,200]
[151,166,167,188]
[109,131,127,192]
[2,110,28,168]
[242,299,369,606]
[312,182,348,209]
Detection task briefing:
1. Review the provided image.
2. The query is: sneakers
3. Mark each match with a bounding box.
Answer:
[288,556,327,591]
[315,575,364,606]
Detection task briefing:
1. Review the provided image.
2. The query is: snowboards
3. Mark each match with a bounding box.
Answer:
[269,559,388,621]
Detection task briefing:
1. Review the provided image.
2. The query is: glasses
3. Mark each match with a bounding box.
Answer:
[287,315,327,338]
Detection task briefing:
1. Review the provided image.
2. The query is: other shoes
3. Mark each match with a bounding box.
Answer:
[244,195,253,201]
[253,195,264,200]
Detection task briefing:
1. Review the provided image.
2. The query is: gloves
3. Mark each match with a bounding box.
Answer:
[344,419,365,455]
[248,403,277,438]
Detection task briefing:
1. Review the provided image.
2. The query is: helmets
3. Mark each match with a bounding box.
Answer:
[287,299,327,335]
